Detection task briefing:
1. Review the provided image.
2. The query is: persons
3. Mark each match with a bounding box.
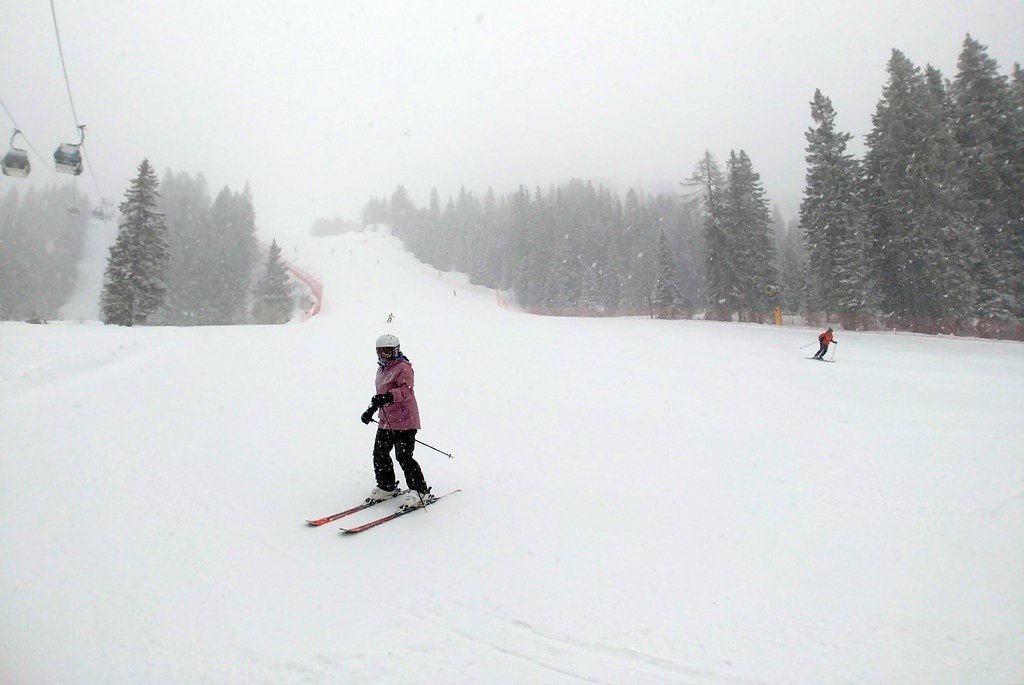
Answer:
[812,329,838,360]
[360,334,434,514]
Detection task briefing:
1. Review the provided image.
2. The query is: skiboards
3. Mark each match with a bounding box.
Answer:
[304,488,462,533]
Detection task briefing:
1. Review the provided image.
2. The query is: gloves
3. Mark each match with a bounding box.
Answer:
[833,341,837,343]
[361,406,378,424]
[372,392,393,408]
[819,338,821,341]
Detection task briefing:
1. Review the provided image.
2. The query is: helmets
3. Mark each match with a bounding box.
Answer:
[376,335,399,362]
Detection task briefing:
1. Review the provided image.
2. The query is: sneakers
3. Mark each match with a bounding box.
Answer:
[364,480,401,503]
[399,487,433,510]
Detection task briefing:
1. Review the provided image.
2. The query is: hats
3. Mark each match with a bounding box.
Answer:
[829,327,833,331]
